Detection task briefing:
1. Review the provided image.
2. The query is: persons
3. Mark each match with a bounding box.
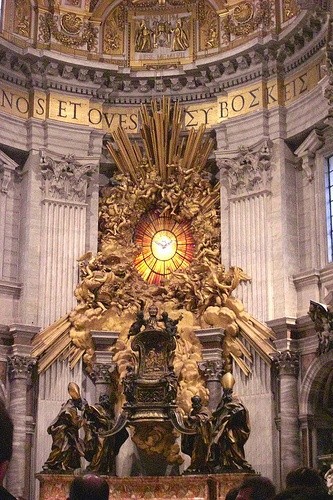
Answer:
[41,167,257,474]
[65,474,109,500]
[225,464,333,500]
[308,304,333,355]
[136,19,190,53]
[0,396,17,500]
